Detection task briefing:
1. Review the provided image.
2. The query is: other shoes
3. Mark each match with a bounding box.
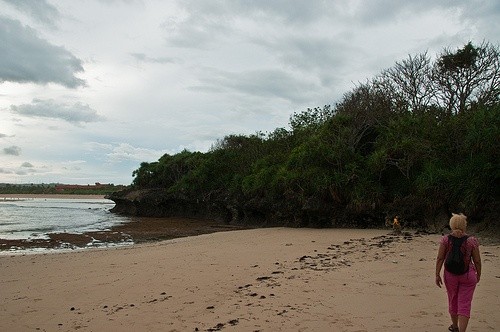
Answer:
[448,325,459,332]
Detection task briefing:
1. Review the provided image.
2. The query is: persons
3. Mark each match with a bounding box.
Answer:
[434,212,482,332]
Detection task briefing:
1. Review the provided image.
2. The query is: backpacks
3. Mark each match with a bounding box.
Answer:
[445,235,474,275]
